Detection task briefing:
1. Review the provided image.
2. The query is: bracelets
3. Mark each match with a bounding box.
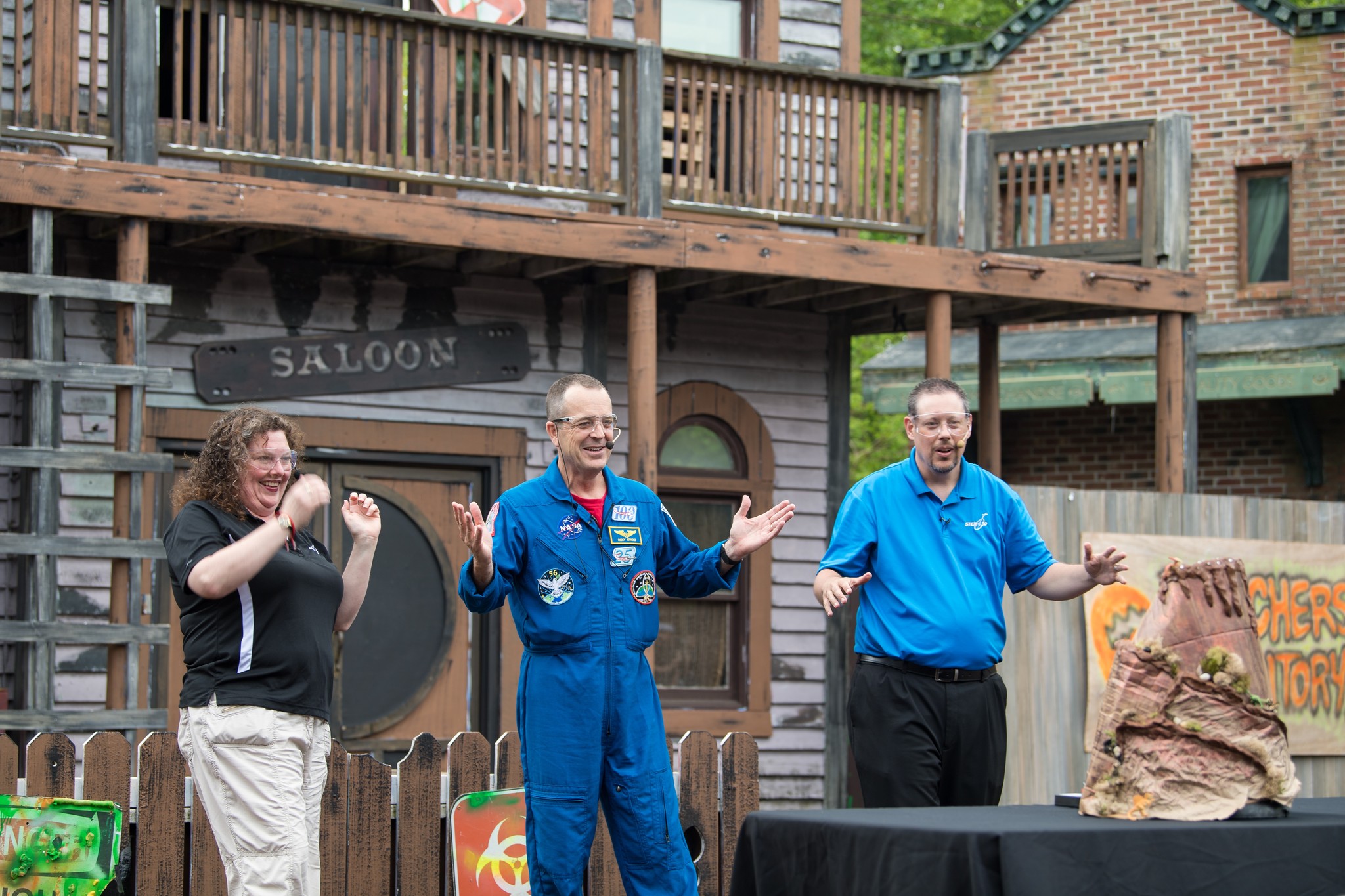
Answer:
[721,540,743,565]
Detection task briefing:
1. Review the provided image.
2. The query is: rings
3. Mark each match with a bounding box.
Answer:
[770,525,774,530]
[1114,564,1119,572]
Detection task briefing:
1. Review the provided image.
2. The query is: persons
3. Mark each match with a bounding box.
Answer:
[166,408,382,896]
[812,378,1128,806]
[452,374,797,896]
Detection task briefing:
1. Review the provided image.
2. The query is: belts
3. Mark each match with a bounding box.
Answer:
[855,653,998,682]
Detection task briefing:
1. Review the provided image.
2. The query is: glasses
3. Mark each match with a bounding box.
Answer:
[242,448,297,471]
[913,412,972,436]
[551,414,618,433]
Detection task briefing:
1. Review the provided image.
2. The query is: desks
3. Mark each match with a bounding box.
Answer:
[725,795,1345,896]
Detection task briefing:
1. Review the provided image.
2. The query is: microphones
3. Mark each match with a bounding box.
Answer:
[291,462,301,480]
[605,428,621,450]
[590,515,598,522]
[956,424,970,448]
[945,518,951,525]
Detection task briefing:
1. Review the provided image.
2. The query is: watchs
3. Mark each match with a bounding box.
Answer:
[276,510,295,536]
